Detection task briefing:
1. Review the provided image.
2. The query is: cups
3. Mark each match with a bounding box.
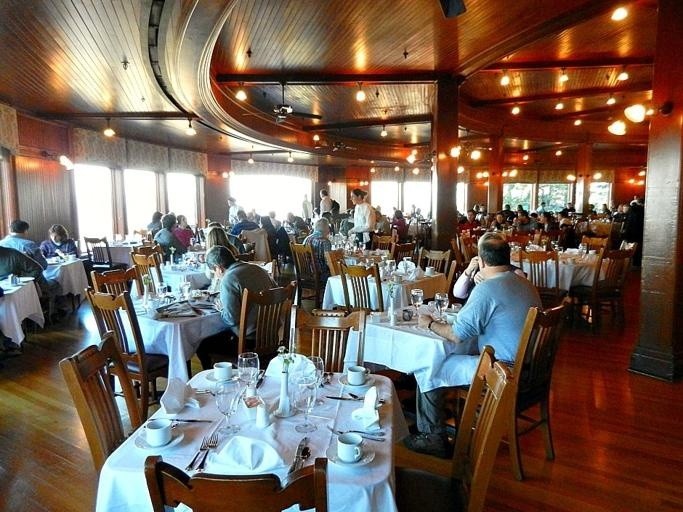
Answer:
[212,361,232,381]
[51,257,59,264]
[551,241,563,255]
[410,289,461,325]
[138,418,172,446]
[359,256,435,284]
[401,309,412,321]
[240,396,265,420]
[7,273,17,287]
[143,282,191,319]
[67,254,75,262]
[370,312,382,323]
[346,366,370,385]
[577,243,596,255]
[336,433,363,462]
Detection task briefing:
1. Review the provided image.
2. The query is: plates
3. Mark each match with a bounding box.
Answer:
[134,427,184,452]
[205,372,236,383]
[47,262,58,265]
[325,444,375,466]
[215,441,279,473]
[17,277,35,283]
[337,375,375,387]
[351,408,378,426]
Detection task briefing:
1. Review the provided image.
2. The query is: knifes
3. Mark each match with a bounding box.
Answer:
[255,371,264,388]
[148,418,211,423]
[285,436,308,474]
[325,395,384,403]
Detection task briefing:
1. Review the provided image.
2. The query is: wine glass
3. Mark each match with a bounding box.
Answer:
[215,380,239,436]
[237,352,259,387]
[328,233,364,255]
[305,356,323,405]
[293,378,317,433]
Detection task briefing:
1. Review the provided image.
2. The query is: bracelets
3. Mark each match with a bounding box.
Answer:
[425,320,434,330]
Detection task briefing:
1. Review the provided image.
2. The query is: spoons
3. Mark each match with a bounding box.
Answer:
[347,392,383,401]
[298,446,310,469]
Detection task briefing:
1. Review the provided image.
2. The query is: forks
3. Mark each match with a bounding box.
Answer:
[326,425,385,442]
[184,436,210,471]
[194,434,217,472]
[317,372,333,389]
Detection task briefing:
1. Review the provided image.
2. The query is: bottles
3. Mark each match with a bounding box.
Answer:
[256,404,269,428]
[243,386,254,399]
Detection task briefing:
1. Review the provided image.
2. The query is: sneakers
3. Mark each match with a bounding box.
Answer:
[402,432,452,458]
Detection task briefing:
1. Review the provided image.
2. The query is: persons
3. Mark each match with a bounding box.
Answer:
[207,197,282,258]
[191,243,281,372]
[0,245,43,282]
[287,188,376,282]
[147,212,195,259]
[401,233,544,458]
[38,222,79,262]
[0,219,49,326]
[459,195,643,266]
[372,204,426,242]
